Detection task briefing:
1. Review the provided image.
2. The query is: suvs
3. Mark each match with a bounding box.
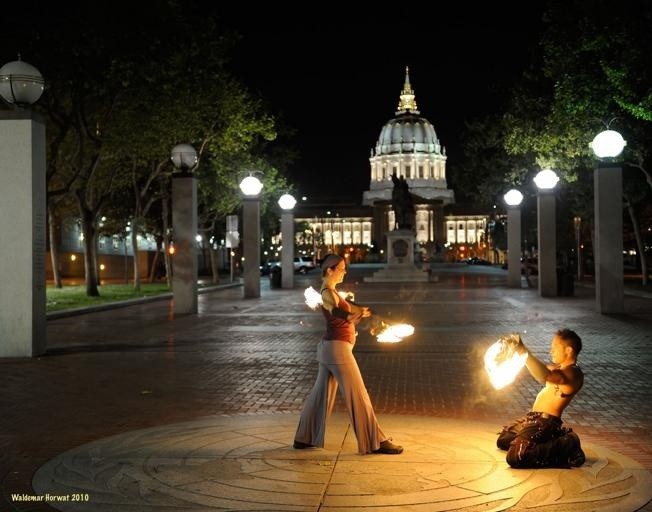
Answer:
[270,255,315,276]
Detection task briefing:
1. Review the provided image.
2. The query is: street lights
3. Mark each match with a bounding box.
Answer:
[278,194,298,290]
[170,144,199,316]
[591,129,627,316]
[238,175,264,299]
[503,188,525,287]
[532,169,561,298]
[0,60,48,358]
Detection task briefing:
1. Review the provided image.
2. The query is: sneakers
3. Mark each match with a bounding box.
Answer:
[565,428,585,467]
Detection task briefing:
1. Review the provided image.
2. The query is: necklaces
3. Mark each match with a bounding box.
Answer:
[554,363,575,393]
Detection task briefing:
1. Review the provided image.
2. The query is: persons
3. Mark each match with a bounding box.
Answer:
[494,325,589,471]
[291,251,405,457]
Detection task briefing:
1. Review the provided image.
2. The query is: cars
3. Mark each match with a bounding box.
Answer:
[519,256,538,276]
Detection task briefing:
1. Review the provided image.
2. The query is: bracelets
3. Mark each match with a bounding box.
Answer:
[330,308,349,322]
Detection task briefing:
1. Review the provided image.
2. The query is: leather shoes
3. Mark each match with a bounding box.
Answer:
[367,440,403,454]
[292,440,317,450]
